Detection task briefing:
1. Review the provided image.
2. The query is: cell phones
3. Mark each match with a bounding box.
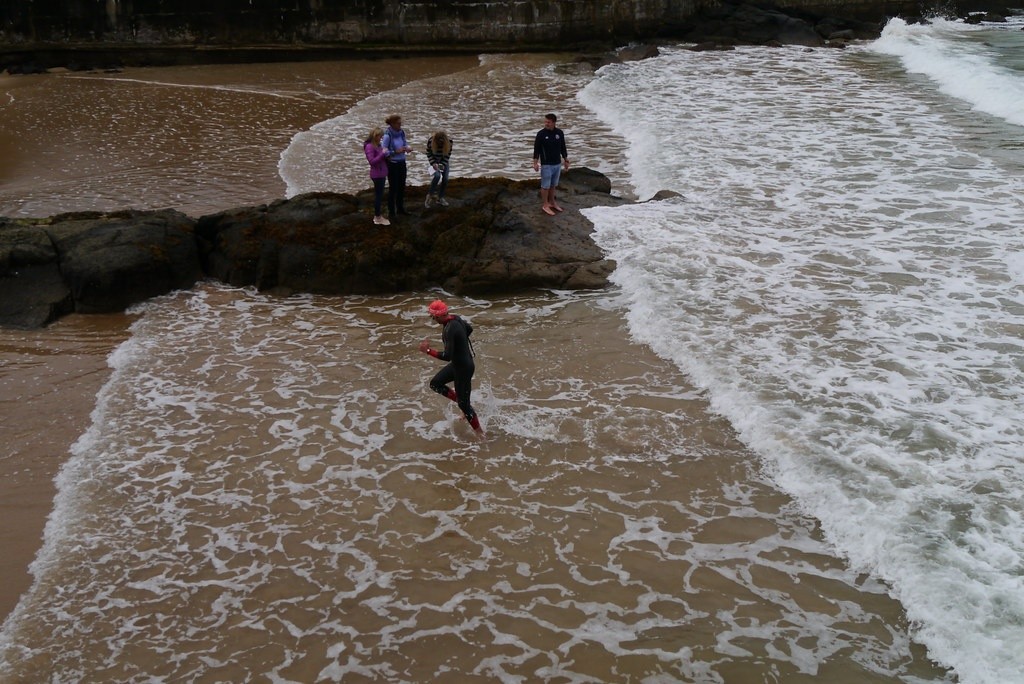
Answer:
[439,165,443,173]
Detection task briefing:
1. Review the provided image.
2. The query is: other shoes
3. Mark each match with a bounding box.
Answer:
[397,206,412,216]
[373,215,390,225]
[388,211,402,222]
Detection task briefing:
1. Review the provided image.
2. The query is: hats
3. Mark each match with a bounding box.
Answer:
[429,299,448,314]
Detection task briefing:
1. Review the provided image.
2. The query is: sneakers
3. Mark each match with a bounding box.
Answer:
[425,193,433,208]
[436,196,449,206]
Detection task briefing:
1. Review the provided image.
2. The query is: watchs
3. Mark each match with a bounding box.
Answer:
[427,349,431,354]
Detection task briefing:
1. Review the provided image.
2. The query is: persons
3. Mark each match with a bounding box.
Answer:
[364,127,390,225]
[383,114,414,219]
[417,300,484,437]
[425,132,453,209]
[533,113,569,215]
